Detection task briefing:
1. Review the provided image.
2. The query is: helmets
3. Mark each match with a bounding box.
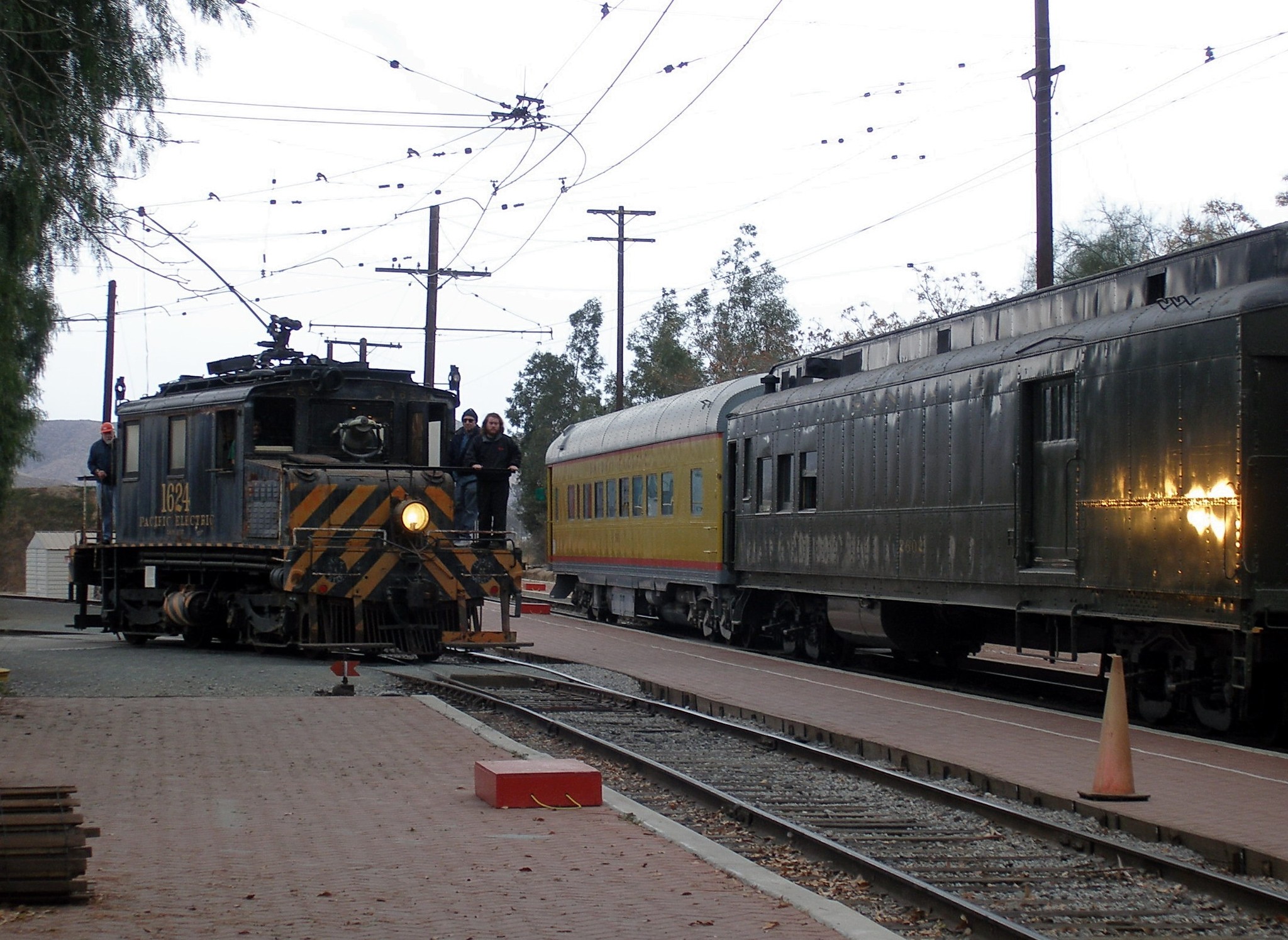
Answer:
[100,422,114,433]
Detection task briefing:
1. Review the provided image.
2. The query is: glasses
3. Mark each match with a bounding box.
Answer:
[463,418,475,422]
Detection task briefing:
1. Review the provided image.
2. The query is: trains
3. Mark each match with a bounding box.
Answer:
[538,218,1288,751]
[67,279,539,659]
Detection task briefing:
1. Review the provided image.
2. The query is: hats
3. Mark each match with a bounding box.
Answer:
[462,408,478,424]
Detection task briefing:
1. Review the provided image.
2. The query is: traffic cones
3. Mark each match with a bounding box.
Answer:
[1074,655,1154,804]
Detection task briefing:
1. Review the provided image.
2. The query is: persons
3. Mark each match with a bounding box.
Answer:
[465,413,521,549]
[227,415,268,465]
[87,422,117,545]
[452,408,482,546]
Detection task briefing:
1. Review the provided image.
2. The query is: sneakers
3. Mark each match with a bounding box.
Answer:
[454,539,473,547]
[489,541,507,549]
[470,540,489,548]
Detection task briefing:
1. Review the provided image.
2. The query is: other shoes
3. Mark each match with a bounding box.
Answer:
[101,536,112,545]
